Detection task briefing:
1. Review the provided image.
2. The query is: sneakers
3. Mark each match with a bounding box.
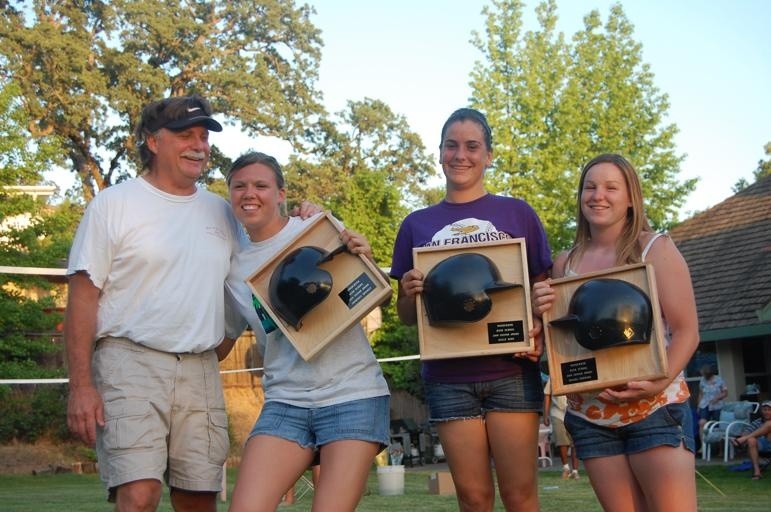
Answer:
[561,468,581,480]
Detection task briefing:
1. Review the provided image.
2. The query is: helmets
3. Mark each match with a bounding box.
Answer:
[544,275,654,352]
[423,251,525,328]
[265,234,353,334]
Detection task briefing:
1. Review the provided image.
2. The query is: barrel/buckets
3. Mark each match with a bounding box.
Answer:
[376,464,405,497]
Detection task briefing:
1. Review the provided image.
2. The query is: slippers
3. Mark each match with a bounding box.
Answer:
[731,437,745,450]
[750,474,761,480]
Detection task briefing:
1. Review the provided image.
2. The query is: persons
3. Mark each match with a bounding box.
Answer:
[542,371,581,481]
[531,152,705,511]
[64,90,327,512]
[387,107,556,510]
[726,398,771,479]
[696,361,731,457]
[210,151,393,512]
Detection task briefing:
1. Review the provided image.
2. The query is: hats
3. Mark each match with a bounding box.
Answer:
[139,94,226,134]
[761,399,771,407]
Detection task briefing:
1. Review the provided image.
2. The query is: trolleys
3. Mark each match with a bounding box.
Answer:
[396,427,448,467]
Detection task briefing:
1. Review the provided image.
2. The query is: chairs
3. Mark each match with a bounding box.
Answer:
[701,400,760,463]
